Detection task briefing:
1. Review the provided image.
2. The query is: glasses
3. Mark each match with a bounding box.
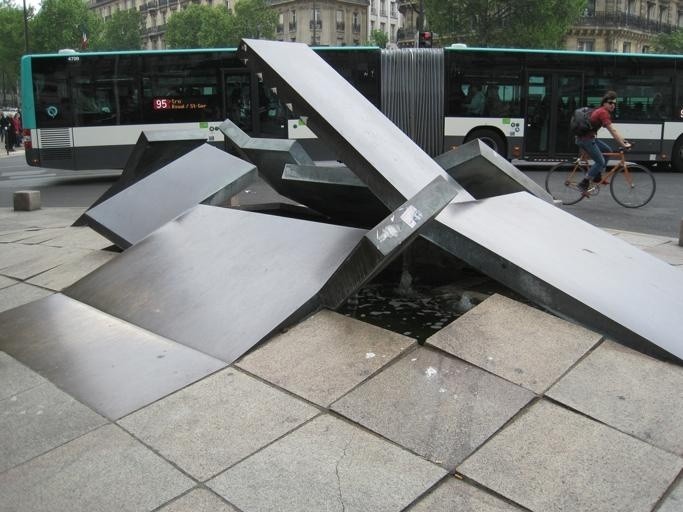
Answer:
[605,101,616,106]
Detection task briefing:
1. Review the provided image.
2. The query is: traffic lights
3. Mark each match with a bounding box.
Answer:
[419,32,431,48]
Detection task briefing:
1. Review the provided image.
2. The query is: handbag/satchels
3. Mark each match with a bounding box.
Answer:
[570,106,595,137]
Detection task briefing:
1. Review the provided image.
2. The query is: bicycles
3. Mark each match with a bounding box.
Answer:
[546,137,655,213]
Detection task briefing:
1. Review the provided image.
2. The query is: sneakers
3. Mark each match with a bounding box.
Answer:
[593,178,610,184]
[576,184,589,196]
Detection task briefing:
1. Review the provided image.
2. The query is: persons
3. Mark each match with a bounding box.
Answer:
[0,107,23,155]
[575,90,632,198]
[72,82,99,115]
[460,83,511,118]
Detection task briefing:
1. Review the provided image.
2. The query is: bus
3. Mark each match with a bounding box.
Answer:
[17,45,683,193]
